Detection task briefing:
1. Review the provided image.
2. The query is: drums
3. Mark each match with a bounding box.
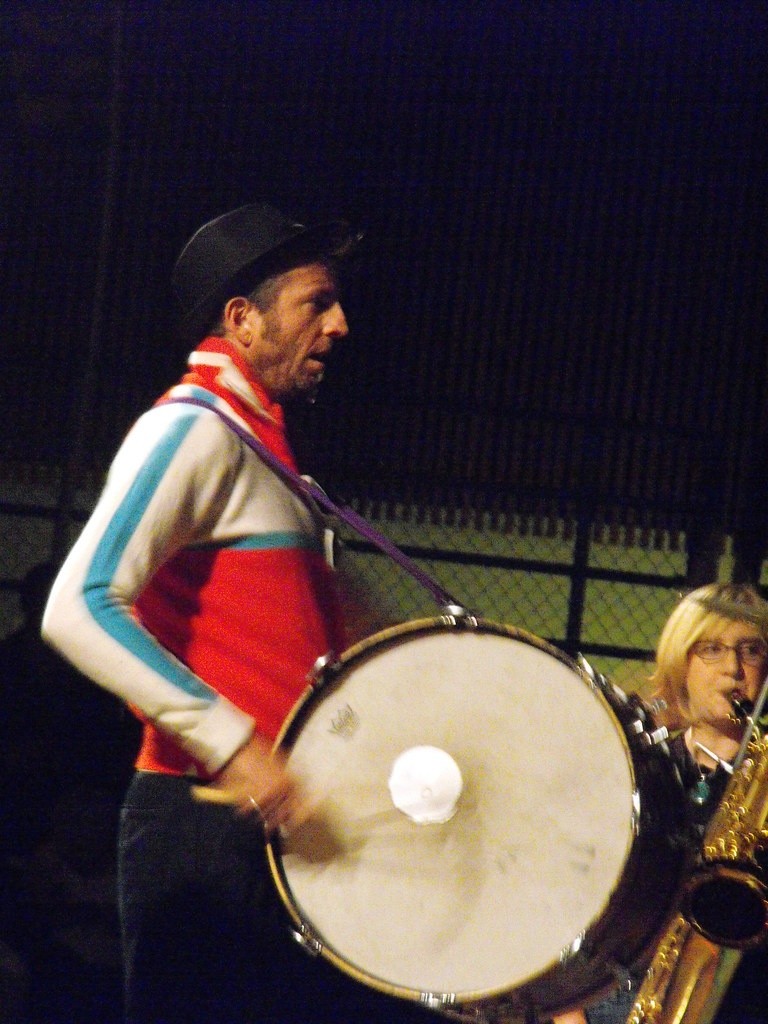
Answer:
[258,608,697,1023]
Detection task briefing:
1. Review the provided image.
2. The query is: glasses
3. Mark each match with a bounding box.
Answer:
[692,639,768,660]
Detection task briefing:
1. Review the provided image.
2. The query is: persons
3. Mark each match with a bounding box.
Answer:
[39,205,353,1024]
[0,205,768,1024]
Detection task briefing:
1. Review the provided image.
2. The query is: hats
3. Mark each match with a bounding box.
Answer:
[167,198,353,338]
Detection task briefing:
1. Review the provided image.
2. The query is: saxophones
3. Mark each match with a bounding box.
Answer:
[624,669,768,1023]
